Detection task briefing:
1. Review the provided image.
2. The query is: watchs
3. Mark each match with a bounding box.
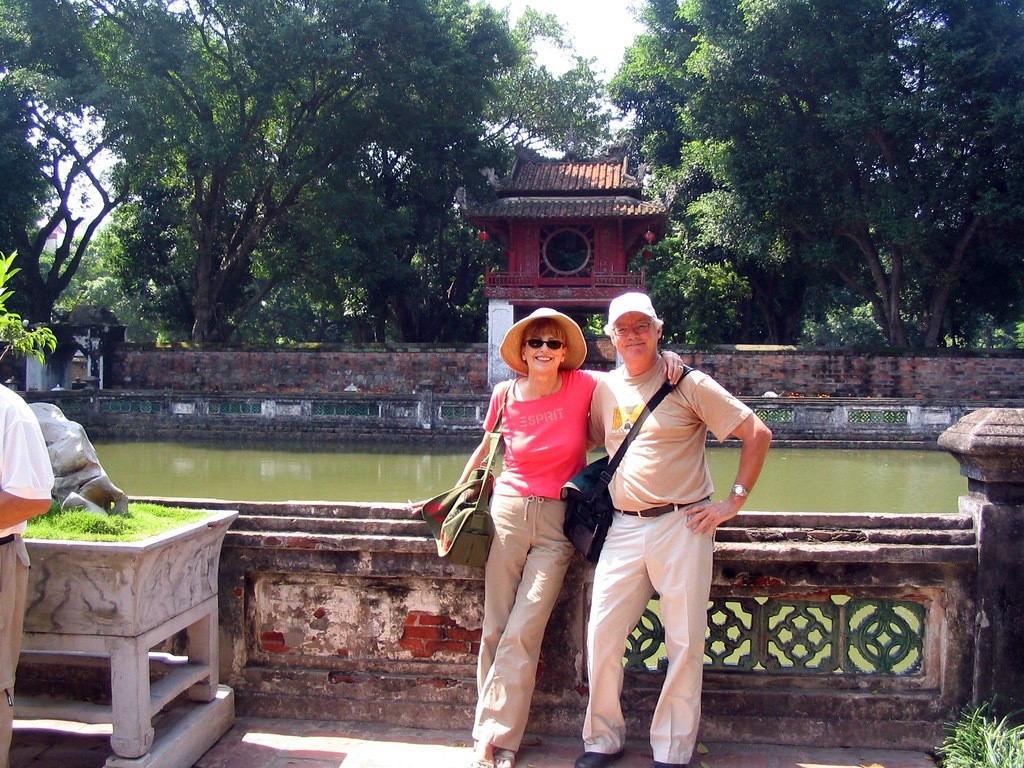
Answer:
[732,485,748,497]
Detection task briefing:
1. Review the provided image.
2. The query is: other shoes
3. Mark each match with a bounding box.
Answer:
[574,749,625,768]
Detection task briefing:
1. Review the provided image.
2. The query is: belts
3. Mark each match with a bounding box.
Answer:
[0,534,14,545]
[613,498,710,517]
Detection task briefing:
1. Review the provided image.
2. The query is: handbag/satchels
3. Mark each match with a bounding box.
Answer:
[422,468,492,566]
[560,456,613,562]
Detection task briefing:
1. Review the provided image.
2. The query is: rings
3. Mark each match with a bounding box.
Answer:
[678,366,683,368]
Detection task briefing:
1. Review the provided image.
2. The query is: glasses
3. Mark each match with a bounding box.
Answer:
[522,338,565,349]
[611,319,656,334]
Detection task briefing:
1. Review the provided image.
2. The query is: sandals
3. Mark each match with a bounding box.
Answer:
[494,752,516,768]
[470,740,494,768]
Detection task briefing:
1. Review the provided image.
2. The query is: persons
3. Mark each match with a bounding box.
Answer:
[0,383,55,768]
[574,292,772,768]
[408,308,683,768]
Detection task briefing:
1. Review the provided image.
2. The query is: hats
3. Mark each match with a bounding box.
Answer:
[499,308,586,376]
[603,292,663,336]
